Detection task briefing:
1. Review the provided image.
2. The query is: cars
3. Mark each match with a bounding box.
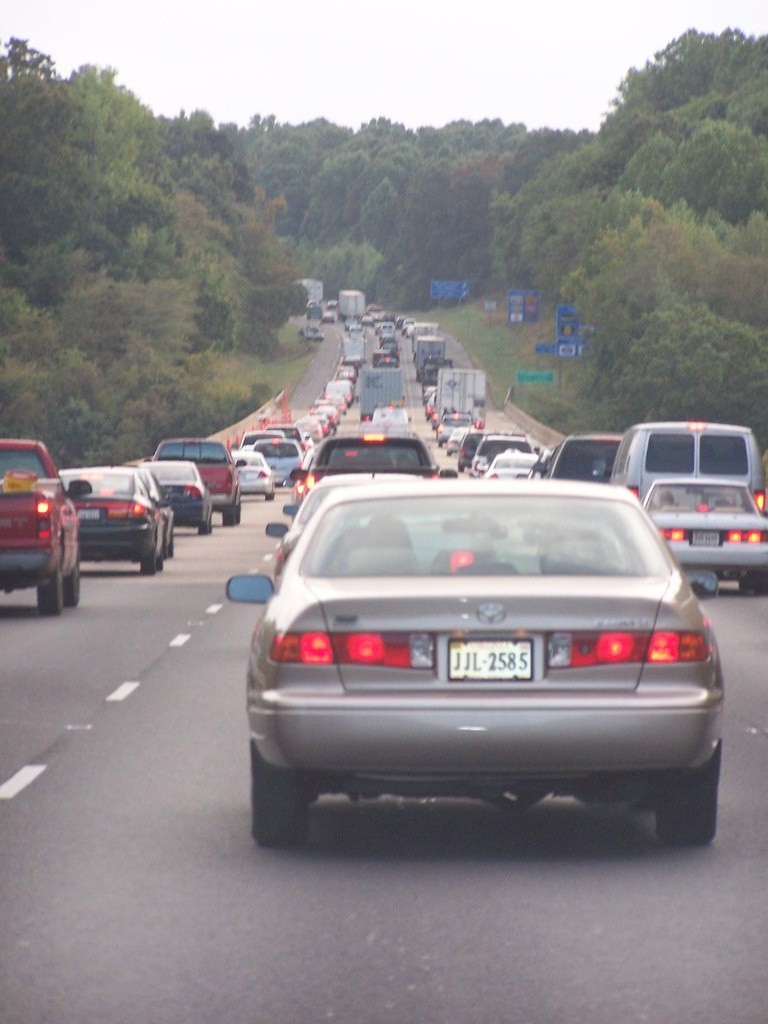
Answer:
[231,449,276,501]
[265,472,501,595]
[55,464,167,577]
[626,478,767,597]
[528,447,551,480]
[238,279,498,485]
[135,459,216,535]
[232,473,728,846]
[121,466,175,558]
[484,449,537,480]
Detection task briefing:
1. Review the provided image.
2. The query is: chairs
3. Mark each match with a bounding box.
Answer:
[328,520,419,575]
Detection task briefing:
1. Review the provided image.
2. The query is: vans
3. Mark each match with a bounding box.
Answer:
[539,433,633,538]
[602,420,766,563]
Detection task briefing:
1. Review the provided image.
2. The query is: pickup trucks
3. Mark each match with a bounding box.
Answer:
[290,436,458,513]
[0,438,94,618]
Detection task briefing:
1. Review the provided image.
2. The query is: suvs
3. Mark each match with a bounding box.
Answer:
[471,433,535,479]
[143,438,248,527]
